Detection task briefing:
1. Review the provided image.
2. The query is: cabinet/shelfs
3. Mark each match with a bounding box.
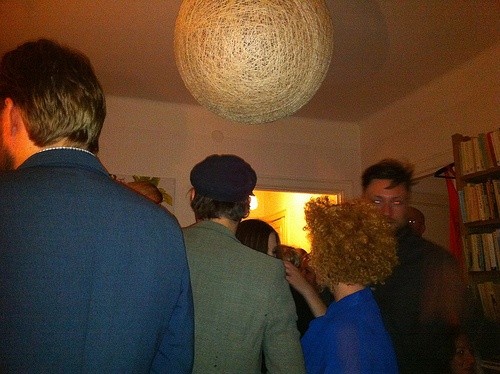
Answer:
[451,133,500,374]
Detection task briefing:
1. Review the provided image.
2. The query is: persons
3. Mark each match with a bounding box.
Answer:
[281,244,325,296]
[448,327,475,374]
[360,158,463,374]
[405,207,426,239]
[235,219,327,318]
[0,37,196,374]
[181,153,307,374]
[300,196,400,374]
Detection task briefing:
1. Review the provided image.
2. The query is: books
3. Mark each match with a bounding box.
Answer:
[460,128,500,322]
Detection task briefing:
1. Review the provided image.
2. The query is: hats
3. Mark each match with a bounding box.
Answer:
[190,154,257,197]
